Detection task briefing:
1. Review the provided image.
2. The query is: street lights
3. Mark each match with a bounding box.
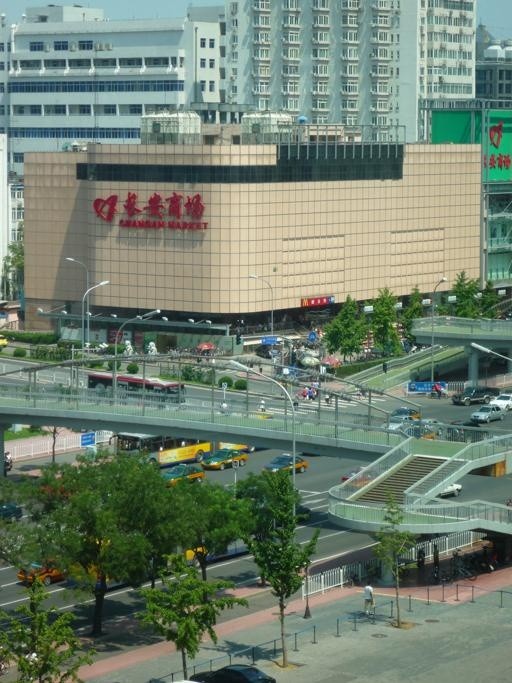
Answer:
[282,365,289,432]
[63,256,92,355]
[112,308,161,401]
[470,341,512,362]
[210,358,215,422]
[80,277,110,388]
[248,274,275,364]
[431,275,448,381]
[228,357,297,519]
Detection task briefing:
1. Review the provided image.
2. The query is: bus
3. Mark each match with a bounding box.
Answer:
[61,534,209,592]
[85,370,187,410]
[105,432,257,467]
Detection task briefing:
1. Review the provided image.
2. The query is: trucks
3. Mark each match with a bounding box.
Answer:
[356,345,376,360]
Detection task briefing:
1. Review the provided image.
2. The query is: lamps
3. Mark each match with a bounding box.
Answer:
[33,303,215,327]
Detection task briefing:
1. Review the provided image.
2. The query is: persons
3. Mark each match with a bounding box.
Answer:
[431,381,449,400]
[299,382,320,401]
[363,581,375,614]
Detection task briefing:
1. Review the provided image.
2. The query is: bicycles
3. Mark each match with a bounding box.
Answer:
[418,541,512,584]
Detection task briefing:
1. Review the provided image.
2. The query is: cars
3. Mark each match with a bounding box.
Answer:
[188,662,278,682]
[470,403,506,423]
[382,403,487,443]
[439,482,464,498]
[489,391,512,411]
[261,449,310,476]
[254,343,283,360]
[0,497,23,525]
[14,558,65,590]
[160,461,206,487]
[340,464,380,488]
[201,444,251,471]
[0,334,9,348]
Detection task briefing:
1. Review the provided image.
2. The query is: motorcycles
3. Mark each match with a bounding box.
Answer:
[430,388,448,399]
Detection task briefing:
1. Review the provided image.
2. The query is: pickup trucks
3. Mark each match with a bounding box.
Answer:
[450,383,501,406]
